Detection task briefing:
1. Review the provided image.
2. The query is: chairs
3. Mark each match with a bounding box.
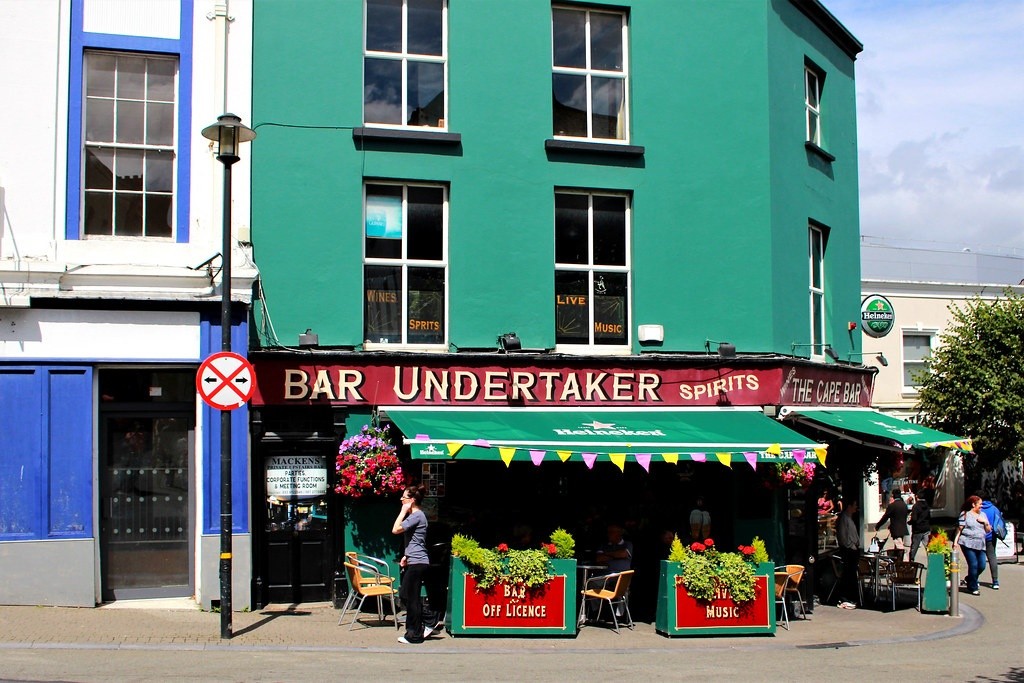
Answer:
[825,546,924,612]
[773,563,806,631]
[337,551,398,631]
[576,569,635,634]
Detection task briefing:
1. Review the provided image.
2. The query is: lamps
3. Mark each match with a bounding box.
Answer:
[299,329,318,347]
[790,343,840,361]
[705,339,737,357]
[499,332,522,354]
[848,352,888,366]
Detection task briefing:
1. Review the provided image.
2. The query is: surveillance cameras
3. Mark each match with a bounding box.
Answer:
[186,250,219,271]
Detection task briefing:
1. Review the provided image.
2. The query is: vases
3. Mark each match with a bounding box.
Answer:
[448,553,578,636]
[656,560,776,637]
[924,552,950,613]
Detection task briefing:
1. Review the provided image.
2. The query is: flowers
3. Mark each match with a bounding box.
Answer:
[670,532,769,605]
[923,529,959,580]
[333,423,407,499]
[776,459,817,495]
[453,529,577,599]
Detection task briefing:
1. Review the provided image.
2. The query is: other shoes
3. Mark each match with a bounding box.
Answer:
[836,599,856,609]
[423,626,433,638]
[398,637,410,643]
[1014,549,1024,555]
[616,605,624,616]
[966,589,980,594]
[993,584,999,588]
[964,577,967,584]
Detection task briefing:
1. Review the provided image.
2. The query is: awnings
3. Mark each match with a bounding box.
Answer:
[783,407,972,448]
[378,406,823,462]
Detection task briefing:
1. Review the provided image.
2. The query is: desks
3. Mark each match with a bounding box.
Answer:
[578,565,608,627]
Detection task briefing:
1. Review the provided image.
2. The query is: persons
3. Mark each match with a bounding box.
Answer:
[392,484,432,644]
[907,490,931,562]
[836,495,860,602]
[584,523,633,628]
[952,495,991,595]
[977,493,1007,589]
[509,525,540,549]
[875,488,910,561]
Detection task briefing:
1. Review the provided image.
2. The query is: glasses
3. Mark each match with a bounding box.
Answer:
[400,496,411,501]
[823,493,828,495]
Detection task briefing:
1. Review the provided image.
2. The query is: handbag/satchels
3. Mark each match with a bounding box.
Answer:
[993,512,1007,541]
